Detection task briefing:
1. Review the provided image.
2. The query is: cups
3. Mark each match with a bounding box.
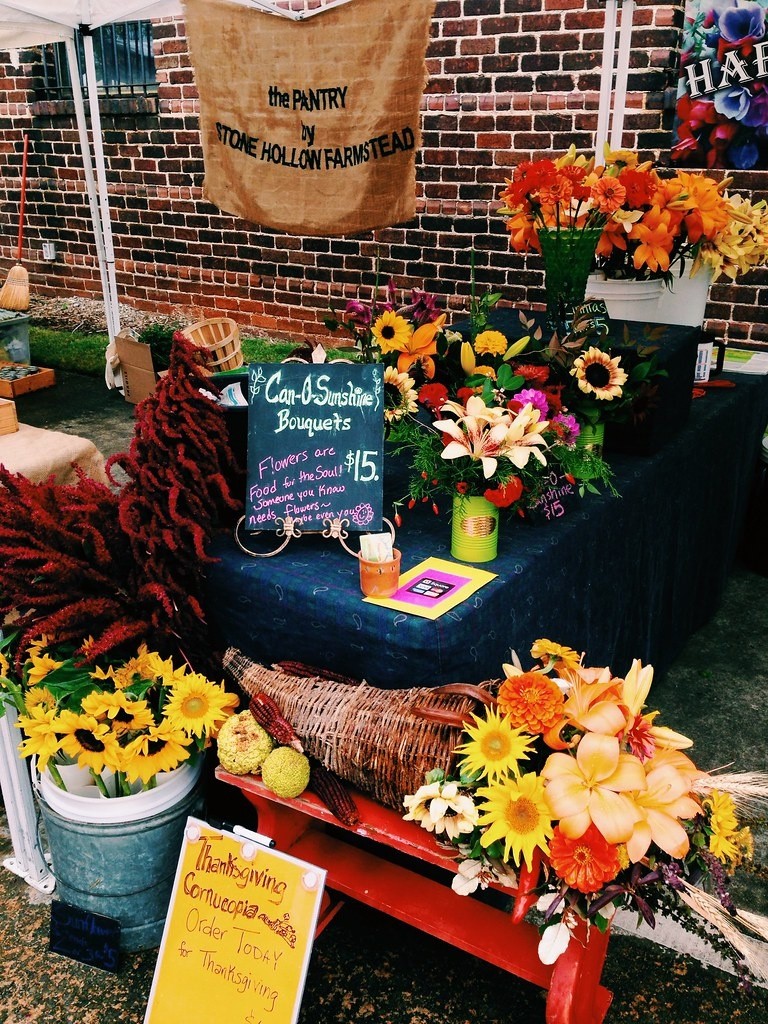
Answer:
[357,547,403,596]
[693,331,725,385]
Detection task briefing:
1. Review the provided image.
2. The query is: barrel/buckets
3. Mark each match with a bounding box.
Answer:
[180,317,243,375]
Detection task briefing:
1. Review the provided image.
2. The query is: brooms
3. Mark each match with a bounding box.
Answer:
[0,133,30,312]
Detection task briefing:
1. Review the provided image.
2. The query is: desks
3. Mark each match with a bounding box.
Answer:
[200,377,767,688]
[213,762,614,1023]
[0,309,30,364]
[0,422,111,490]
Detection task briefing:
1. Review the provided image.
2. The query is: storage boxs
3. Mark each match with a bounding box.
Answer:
[0,360,56,398]
[114,333,174,408]
[0,398,18,436]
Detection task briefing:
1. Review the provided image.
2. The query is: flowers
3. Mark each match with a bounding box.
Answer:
[0,635,239,797]
[489,145,656,258]
[673,192,768,282]
[567,167,754,296]
[0,334,251,671]
[282,243,665,534]
[399,639,756,965]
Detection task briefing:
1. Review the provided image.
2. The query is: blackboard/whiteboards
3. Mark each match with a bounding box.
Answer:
[246,362,385,531]
[50,899,122,976]
[144,816,328,1024]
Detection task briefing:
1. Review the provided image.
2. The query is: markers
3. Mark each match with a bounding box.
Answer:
[221,821,275,848]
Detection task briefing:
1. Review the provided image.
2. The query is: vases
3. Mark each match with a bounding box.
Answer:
[537,225,601,337]
[561,422,606,481]
[654,242,714,326]
[584,275,667,326]
[451,492,497,562]
